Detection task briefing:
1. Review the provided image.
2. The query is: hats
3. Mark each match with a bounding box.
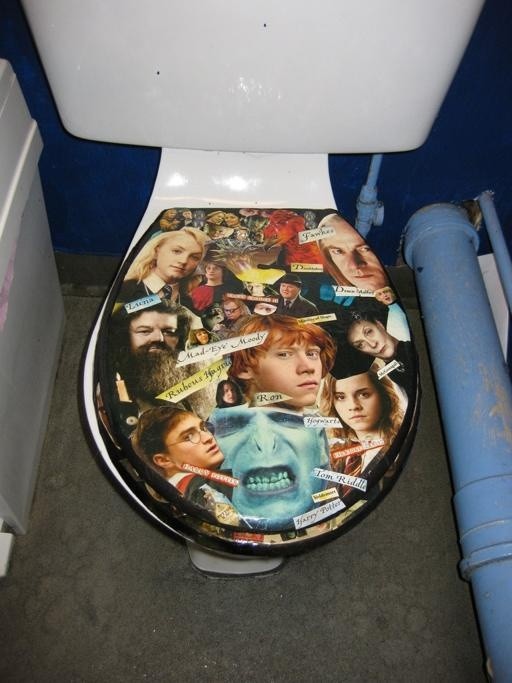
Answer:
[278,273,302,288]
[317,296,389,381]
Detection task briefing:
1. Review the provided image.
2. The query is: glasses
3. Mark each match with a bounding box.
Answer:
[167,422,207,445]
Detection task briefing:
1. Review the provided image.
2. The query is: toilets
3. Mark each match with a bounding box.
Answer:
[25,0,481,578]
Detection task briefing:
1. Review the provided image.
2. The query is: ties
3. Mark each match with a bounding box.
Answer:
[342,441,362,498]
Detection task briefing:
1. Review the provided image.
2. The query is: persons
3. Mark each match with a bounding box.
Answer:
[95,199,414,541]
